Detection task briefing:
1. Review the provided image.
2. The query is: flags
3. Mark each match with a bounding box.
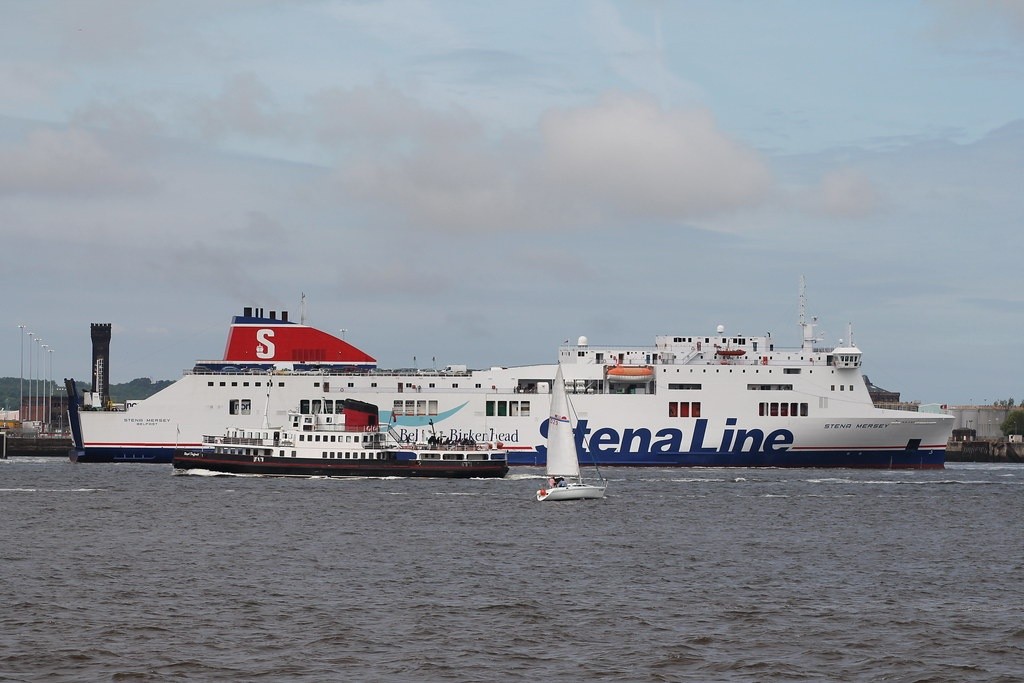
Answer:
[391,411,397,422]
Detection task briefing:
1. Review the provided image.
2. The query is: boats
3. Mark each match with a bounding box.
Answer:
[172,368,509,478]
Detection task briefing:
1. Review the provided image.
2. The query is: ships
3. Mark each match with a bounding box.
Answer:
[64,275,956,470]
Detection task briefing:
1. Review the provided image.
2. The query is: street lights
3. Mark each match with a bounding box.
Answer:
[19,326,27,424]
[48,350,54,435]
[34,338,42,424]
[41,345,48,423]
[27,331,35,421]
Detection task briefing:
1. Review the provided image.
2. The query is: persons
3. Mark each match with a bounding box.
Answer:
[549,476,557,488]
[405,435,413,445]
[560,478,566,487]
[427,435,476,450]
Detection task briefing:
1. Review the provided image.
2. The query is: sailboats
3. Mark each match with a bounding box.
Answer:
[536,361,608,501]
